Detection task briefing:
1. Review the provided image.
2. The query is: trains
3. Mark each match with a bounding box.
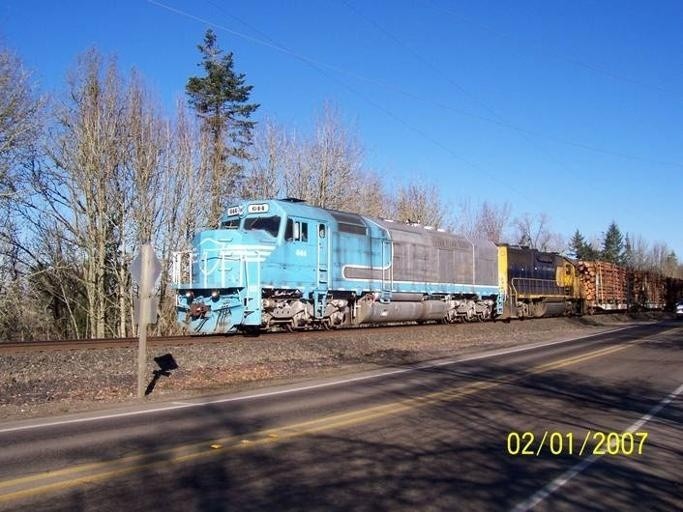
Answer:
[166,195,683,337]
